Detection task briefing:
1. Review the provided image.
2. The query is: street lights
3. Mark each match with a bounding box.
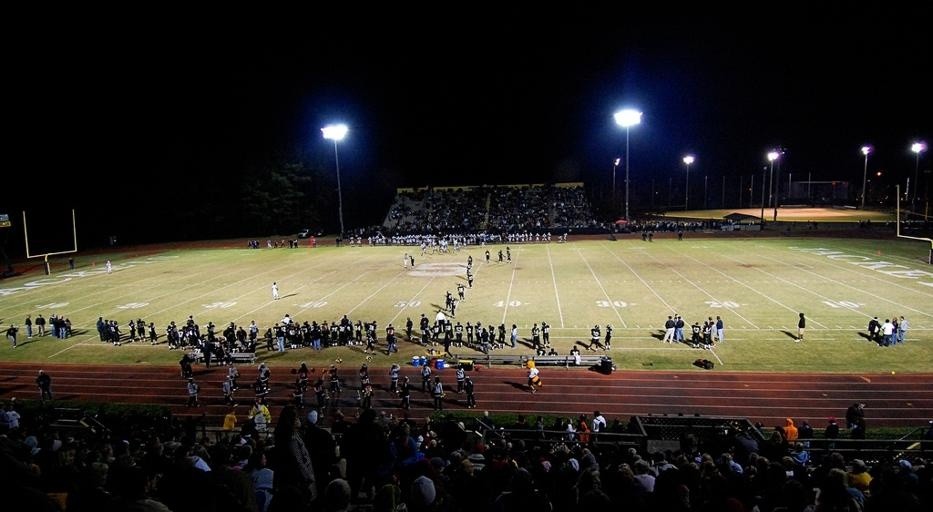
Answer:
[318,122,354,236]
[610,154,623,201]
[611,108,643,221]
[859,145,873,210]
[907,140,927,197]
[680,153,696,210]
[764,149,777,209]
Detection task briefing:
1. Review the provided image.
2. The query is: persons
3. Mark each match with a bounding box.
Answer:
[868,315,908,347]
[336,185,737,255]
[445,255,474,318]
[1,396,933,511]
[486,247,513,263]
[888,220,931,238]
[697,359,713,369]
[692,316,723,350]
[272,282,279,299]
[68,257,75,270]
[662,314,685,343]
[793,313,805,342]
[5,308,616,419]
[248,235,317,249]
[403,253,415,268]
[106,259,112,273]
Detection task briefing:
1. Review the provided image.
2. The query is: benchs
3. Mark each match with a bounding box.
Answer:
[454,354,607,369]
[191,352,256,364]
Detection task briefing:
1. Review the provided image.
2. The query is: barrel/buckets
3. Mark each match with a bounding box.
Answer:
[411,355,445,370]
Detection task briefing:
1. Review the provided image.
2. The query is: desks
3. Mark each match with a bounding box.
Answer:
[424,351,447,360]
